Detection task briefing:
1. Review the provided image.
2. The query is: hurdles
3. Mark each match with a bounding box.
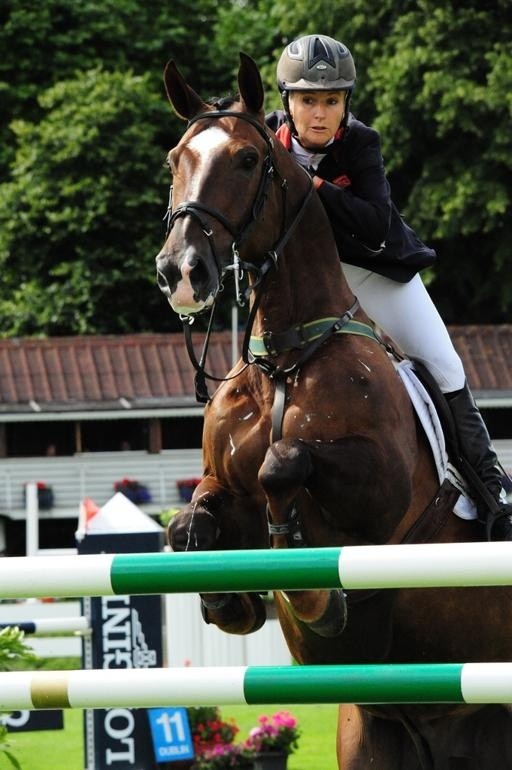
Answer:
[1,533,511,710]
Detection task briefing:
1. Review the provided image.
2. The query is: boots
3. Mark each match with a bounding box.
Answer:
[443,376,512,541]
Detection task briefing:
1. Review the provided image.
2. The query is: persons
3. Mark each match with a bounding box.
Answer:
[264,33,512,542]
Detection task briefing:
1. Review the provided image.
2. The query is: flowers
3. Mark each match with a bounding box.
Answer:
[185,703,300,770]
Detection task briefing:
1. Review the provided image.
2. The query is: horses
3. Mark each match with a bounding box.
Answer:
[147,50,511,770]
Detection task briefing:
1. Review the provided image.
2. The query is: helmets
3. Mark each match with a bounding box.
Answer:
[276,34,356,93]
